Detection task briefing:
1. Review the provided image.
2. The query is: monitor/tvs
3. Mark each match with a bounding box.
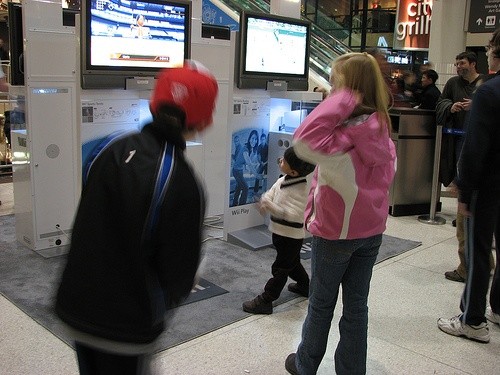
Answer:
[7,2,80,86]
[237,10,311,91]
[202,24,230,40]
[80,0,192,90]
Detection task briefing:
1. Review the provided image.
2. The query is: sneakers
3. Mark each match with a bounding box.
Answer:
[285,353,298,375]
[437,314,490,343]
[288,283,309,296]
[445,270,467,282]
[484,306,500,326]
[242,295,273,314]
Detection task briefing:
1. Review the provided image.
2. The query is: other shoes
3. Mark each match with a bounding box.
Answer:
[452,219,456,226]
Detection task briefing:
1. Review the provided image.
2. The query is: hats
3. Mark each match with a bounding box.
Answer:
[151,61,218,132]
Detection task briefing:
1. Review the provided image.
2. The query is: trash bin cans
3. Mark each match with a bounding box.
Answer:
[388,108,441,216]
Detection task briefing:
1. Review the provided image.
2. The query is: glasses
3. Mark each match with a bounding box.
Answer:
[454,61,471,66]
[485,45,495,50]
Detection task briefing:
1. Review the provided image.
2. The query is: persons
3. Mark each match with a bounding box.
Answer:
[285,52,396,375]
[232,135,242,162]
[404,70,441,108]
[437,29,500,344]
[53,60,219,375]
[4,95,25,146]
[233,130,264,206]
[438,53,486,225]
[444,74,495,283]
[132,14,152,39]
[254,133,268,192]
[242,147,315,315]
